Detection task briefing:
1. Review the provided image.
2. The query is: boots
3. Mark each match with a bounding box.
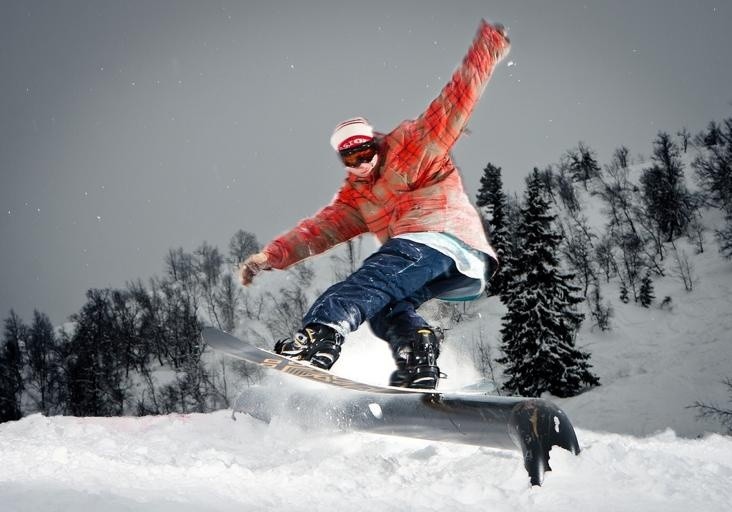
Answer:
[387,331,442,389]
[275,324,345,371]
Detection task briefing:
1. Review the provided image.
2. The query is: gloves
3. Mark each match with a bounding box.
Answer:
[238,252,269,288]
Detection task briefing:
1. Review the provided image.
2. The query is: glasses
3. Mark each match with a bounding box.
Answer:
[337,139,376,168]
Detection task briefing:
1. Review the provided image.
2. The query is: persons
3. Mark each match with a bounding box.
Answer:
[241,16,511,389]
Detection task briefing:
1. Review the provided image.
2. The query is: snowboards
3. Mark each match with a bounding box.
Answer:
[201,326,499,395]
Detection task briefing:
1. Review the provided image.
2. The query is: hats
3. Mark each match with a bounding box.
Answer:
[329,117,378,155]
